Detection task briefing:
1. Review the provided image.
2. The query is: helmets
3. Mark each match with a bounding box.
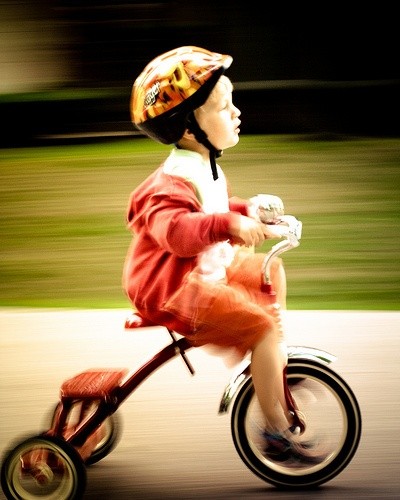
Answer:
[130,46,233,146]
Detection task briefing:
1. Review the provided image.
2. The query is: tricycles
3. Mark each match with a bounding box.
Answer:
[0,207,363,499]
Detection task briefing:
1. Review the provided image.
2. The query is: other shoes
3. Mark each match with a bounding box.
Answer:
[260,423,330,465]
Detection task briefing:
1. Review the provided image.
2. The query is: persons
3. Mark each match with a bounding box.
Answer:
[123,46,335,468]
